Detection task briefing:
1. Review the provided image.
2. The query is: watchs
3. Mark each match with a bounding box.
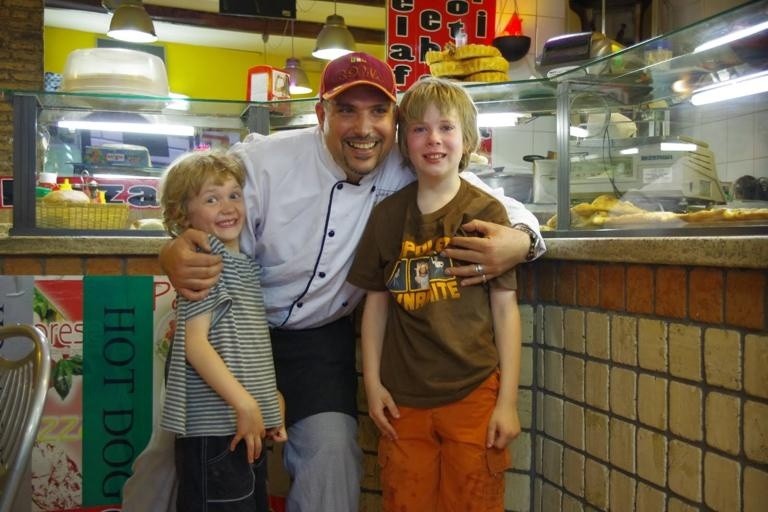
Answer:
[516,225,542,260]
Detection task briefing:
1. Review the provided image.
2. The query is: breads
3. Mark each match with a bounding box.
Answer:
[43,189,91,200]
[548,192,768,230]
[425,43,512,83]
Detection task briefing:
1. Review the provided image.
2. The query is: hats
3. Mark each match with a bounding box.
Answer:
[320,52,398,104]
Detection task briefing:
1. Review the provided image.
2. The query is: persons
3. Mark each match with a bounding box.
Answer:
[155,148,293,512]
[343,74,524,511]
[117,49,549,512]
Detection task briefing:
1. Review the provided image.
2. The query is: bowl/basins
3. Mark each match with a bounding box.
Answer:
[480,174,533,204]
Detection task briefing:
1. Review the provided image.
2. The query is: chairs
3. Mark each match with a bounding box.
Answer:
[0,323,50,512]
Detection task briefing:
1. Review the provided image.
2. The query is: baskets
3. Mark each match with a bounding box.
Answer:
[34,198,129,231]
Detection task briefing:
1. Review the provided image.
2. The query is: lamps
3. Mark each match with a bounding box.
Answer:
[689,70,768,105]
[52,119,203,137]
[471,111,520,129]
[311,0,357,60]
[106,0,159,43]
[281,19,313,94]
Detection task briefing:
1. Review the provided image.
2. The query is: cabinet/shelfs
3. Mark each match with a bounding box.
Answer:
[2,77,563,235]
[559,0,768,236]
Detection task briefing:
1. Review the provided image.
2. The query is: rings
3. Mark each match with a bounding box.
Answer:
[481,274,487,283]
[475,264,484,274]
